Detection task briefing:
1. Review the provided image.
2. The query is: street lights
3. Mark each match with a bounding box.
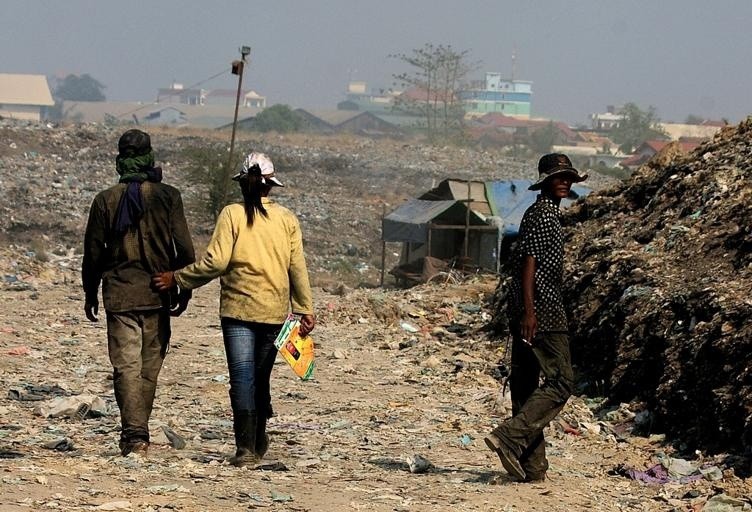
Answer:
[222,46,251,206]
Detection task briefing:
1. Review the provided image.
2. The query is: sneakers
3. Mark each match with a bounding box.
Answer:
[230,433,269,470]
[120,438,148,458]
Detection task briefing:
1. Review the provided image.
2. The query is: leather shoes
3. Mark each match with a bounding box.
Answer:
[484,432,526,480]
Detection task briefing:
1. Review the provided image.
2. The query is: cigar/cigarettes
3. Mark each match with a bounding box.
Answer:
[522,338,533,347]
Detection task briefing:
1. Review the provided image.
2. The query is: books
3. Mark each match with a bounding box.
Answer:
[274,312,315,382]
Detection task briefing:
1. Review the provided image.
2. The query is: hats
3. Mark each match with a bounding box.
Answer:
[232,153,284,187]
[528,154,587,191]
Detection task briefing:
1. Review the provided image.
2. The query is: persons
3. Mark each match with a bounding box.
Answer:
[151,150,315,469]
[484,153,589,484]
[80,130,195,456]
